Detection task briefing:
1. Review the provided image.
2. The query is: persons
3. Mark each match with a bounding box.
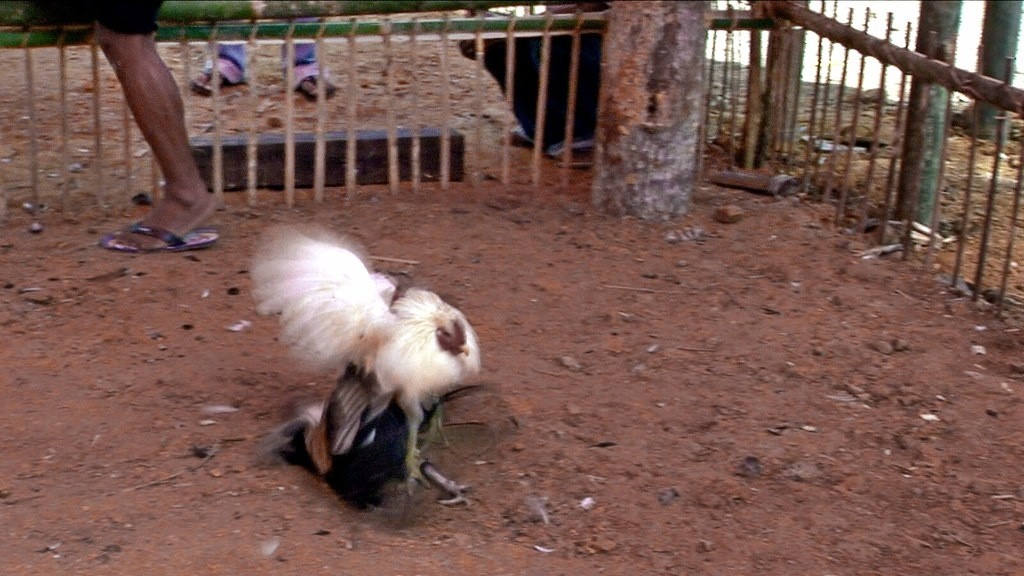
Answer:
[458,0,611,166]
[30,0,220,255]
[190,0,339,102]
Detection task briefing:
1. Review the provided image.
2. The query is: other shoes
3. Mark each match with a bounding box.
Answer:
[500,125,533,148]
[546,137,596,158]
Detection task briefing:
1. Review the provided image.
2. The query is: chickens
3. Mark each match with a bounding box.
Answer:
[250,222,479,513]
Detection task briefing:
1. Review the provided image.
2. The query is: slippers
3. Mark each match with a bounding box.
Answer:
[98,220,219,253]
[191,67,248,96]
[294,75,336,102]
